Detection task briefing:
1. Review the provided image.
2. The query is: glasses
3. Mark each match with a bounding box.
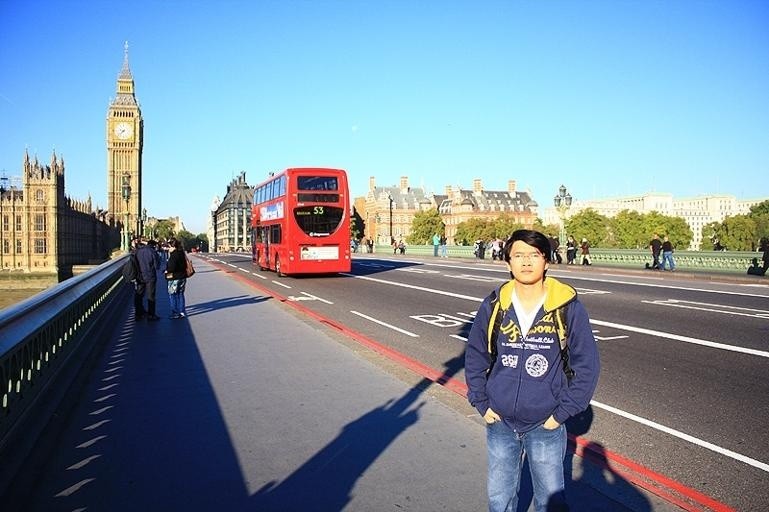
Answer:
[506,253,547,263]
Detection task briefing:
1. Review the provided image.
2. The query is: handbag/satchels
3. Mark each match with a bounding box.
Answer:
[164,273,174,279]
[184,257,194,278]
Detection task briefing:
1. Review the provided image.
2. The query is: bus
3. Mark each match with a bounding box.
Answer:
[250,166,352,279]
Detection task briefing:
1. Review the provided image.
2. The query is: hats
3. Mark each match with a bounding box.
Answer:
[581,238,586,242]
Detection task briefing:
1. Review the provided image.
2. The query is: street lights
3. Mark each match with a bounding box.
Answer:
[554,184,574,246]
[142,207,147,237]
[386,192,393,237]
[120,169,133,254]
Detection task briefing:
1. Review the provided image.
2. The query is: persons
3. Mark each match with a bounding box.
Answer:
[650,235,664,271]
[660,237,676,272]
[130,238,195,321]
[465,230,600,512]
[347,232,592,265]
[756,238,769,275]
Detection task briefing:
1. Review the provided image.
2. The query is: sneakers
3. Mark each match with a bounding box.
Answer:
[650,267,676,273]
[133,313,185,321]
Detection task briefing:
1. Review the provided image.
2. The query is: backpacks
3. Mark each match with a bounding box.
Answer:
[121,248,142,290]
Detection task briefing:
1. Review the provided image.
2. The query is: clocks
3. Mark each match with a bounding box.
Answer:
[114,121,134,140]
